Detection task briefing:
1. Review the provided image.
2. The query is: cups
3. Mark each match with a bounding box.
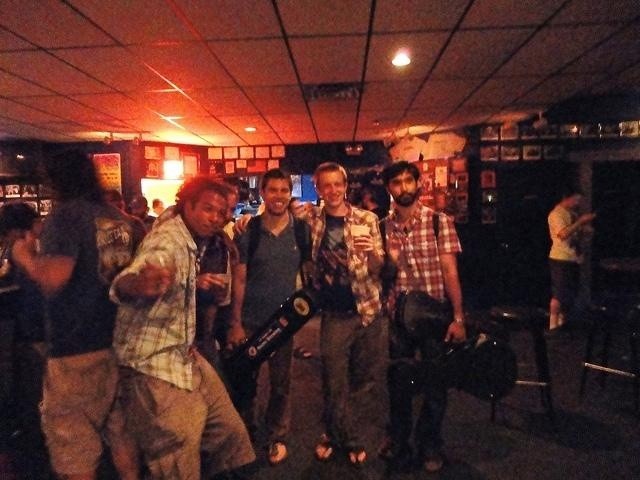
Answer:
[213,274,232,306]
[144,251,175,289]
[352,226,369,249]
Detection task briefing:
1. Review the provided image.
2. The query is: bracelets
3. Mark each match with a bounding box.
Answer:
[454,318,464,323]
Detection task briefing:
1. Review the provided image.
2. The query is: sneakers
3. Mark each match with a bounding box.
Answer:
[265,430,447,472]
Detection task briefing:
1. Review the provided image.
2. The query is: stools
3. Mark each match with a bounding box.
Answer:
[477,303,552,426]
[581,256,640,415]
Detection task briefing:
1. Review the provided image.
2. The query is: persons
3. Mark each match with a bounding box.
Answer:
[543,181,598,342]
[232,160,387,470]
[374,162,467,472]
[1,147,143,479]
[359,185,398,302]
[129,196,158,232]
[110,173,258,479]
[193,167,316,465]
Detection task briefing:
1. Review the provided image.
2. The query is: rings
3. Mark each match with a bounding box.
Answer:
[156,284,160,288]
